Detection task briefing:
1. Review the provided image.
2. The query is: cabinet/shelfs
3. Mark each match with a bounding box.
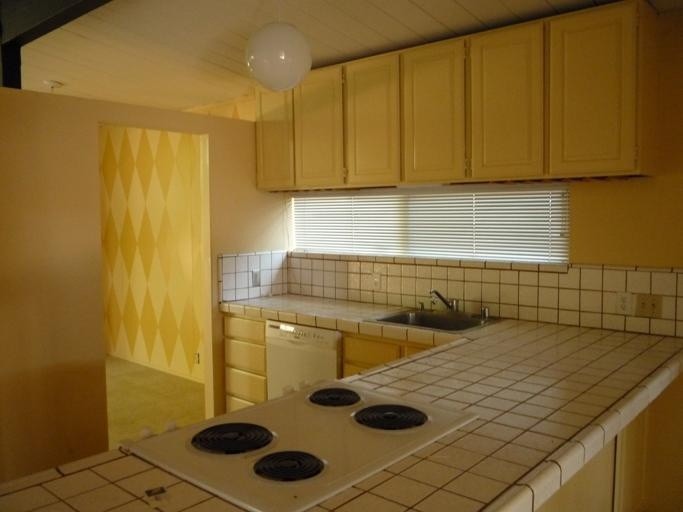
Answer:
[259,64,345,189]
[0,314,683,512]
[472,1,637,180]
[345,34,468,187]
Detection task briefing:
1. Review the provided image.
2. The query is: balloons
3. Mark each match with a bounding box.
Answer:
[243,19,312,94]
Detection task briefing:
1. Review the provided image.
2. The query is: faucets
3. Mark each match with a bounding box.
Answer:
[429,289,459,314]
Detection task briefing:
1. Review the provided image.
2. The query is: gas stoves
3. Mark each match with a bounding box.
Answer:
[125,379,478,512]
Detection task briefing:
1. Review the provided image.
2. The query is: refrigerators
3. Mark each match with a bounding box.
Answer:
[264,318,342,403]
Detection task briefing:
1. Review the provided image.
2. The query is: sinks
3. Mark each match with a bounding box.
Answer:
[362,308,503,336]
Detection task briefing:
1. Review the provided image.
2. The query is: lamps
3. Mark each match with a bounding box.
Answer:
[243,8,313,90]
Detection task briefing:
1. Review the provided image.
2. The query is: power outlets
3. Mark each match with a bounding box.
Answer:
[252,268,261,286]
[613,289,663,320]
